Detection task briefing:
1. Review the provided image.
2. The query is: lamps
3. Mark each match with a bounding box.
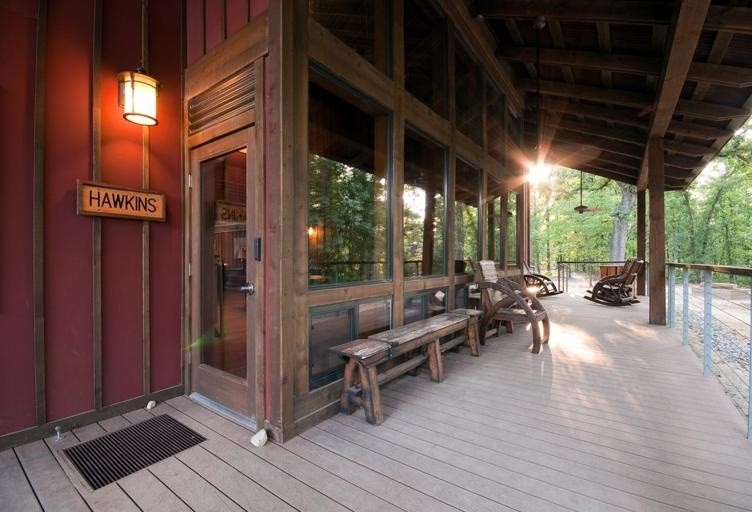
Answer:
[116,64,161,130]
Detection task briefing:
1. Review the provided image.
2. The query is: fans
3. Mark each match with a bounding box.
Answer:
[560,166,605,218]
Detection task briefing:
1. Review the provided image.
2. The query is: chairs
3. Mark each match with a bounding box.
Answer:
[521,260,564,297]
[469,256,551,354]
[584,258,646,307]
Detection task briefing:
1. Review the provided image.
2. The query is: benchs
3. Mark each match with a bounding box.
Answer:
[326,307,486,427]
